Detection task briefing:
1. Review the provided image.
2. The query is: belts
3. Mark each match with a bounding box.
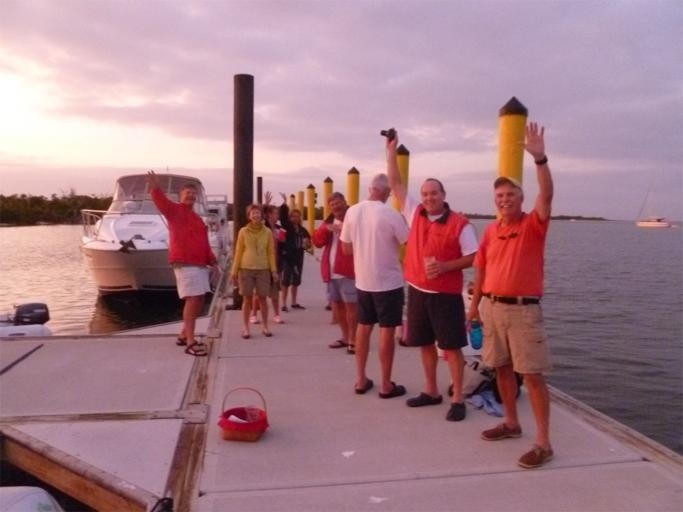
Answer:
[488,295,538,305]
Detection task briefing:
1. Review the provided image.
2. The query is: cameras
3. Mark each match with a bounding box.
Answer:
[381,129,395,139]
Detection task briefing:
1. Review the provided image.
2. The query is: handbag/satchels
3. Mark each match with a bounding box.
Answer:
[448,359,494,398]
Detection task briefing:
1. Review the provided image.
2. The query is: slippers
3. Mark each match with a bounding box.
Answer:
[379,382,405,398]
[406,392,442,407]
[355,378,373,394]
[446,402,466,421]
[329,340,347,348]
[348,344,354,354]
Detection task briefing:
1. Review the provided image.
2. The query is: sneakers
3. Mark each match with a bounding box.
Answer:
[517,445,553,468]
[242,304,306,339]
[481,423,521,440]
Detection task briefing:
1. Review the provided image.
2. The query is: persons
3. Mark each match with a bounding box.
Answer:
[341,172,407,398]
[386,129,480,421]
[233,191,312,339]
[312,191,359,352]
[462,119,557,467]
[143,168,221,357]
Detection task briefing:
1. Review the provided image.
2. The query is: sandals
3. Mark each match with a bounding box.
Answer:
[176,338,205,345]
[185,341,208,356]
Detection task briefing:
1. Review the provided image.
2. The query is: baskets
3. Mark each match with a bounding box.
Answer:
[221,387,267,441]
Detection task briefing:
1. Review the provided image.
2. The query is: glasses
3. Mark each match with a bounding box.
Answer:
[497,232,517,240]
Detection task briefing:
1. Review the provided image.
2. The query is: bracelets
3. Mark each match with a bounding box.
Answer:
[534,155,549,167]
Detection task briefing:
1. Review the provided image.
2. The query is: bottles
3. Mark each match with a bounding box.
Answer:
[467,317,484,351]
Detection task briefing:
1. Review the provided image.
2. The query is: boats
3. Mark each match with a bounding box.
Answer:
[80,168,228,291]
[635,216,670,228]
[1,301,51,336]
[88,292,212,335]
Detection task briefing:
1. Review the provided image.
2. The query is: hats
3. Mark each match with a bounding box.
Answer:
[492,176,522,189]
